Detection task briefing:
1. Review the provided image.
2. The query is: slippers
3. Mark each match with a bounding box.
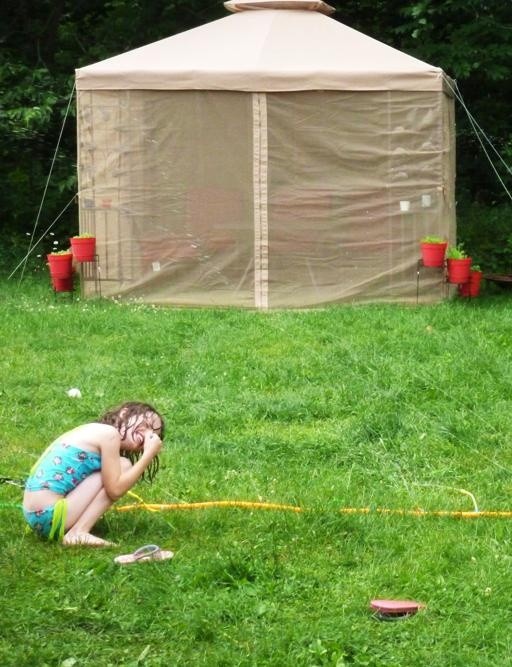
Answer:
[112,541,175,563]
[367,598,428,621]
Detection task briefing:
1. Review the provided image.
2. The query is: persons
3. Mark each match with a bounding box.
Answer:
[23,400,168,546]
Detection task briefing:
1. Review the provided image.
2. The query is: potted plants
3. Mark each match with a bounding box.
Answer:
[419,234,483,298]
[47,233,96,293]
